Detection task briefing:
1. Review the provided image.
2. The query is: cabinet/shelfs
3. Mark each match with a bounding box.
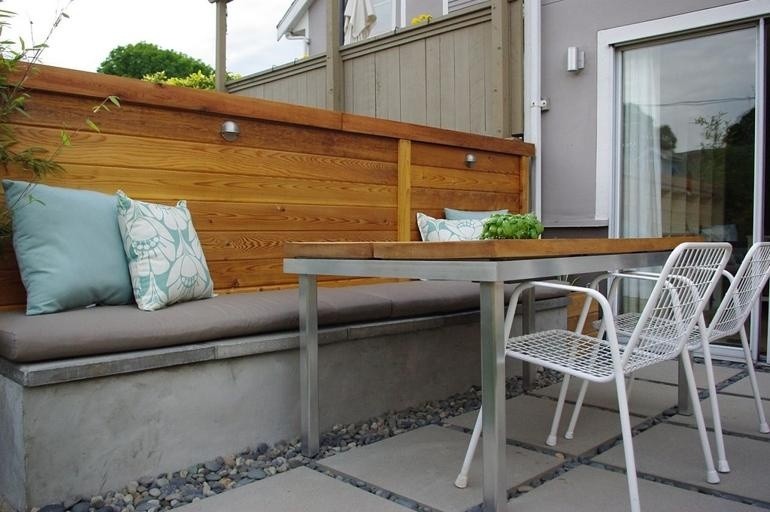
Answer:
[660,88,727,237]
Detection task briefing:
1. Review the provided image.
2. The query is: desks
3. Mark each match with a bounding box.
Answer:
[280,236,709,512]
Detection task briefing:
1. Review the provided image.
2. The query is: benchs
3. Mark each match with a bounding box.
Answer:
[1,60,538,511]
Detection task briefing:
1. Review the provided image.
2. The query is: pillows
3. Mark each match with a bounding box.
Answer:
[414,211,492,241]
[441,206,509,222]
[115,185,214,312]
[1,178,134,317]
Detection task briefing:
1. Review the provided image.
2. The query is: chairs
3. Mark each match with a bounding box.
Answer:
[451,242,734,512]
[564,241,770,474]
